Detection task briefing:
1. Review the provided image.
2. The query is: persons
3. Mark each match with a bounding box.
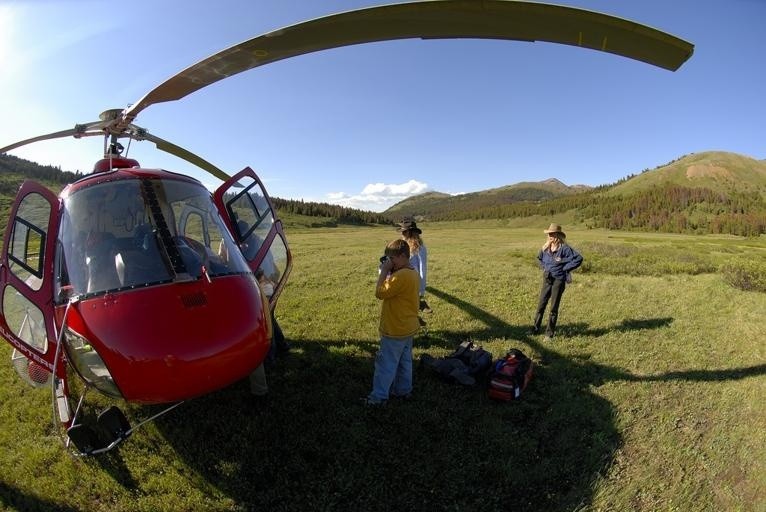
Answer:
[358,240,422,405]
[523,223,584,338]
[218,220,290,365]
[394,220,428,299]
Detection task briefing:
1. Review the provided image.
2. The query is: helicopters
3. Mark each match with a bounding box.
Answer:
[2,0,699,475]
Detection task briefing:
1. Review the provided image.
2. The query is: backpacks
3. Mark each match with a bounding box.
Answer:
[486,348,533,402]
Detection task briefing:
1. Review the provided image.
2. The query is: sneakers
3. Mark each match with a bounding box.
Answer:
[525,328,535,335]
[542,337,551,342]
[359,396,386,409]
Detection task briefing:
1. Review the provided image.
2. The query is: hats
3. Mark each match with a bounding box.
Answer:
[543,222,566,239]
[396,219,422,234]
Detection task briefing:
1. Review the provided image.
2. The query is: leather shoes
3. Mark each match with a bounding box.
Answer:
[417,315,427,326]
[419,299,433,313]
